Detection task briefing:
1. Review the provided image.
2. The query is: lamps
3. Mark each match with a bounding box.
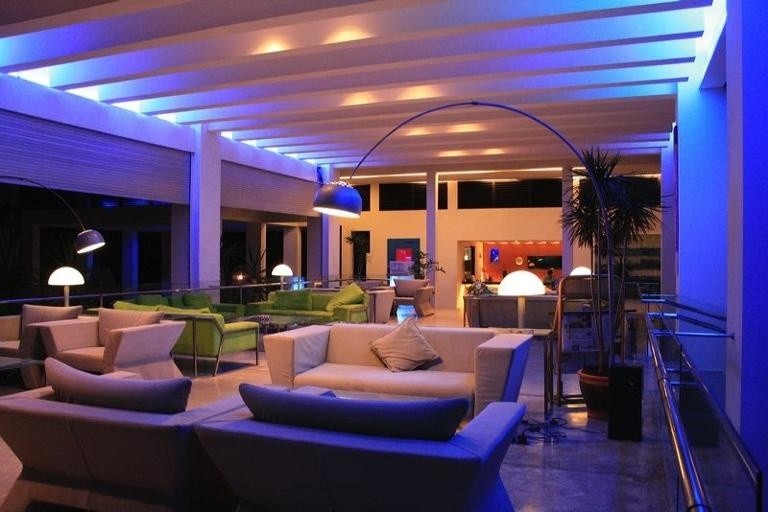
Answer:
[312,101,618,375]
[0,174,106,254]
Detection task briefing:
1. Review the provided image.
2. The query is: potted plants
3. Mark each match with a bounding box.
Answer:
[552,144,674,418]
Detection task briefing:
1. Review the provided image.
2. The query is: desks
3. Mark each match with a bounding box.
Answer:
[486,325,554,420]
